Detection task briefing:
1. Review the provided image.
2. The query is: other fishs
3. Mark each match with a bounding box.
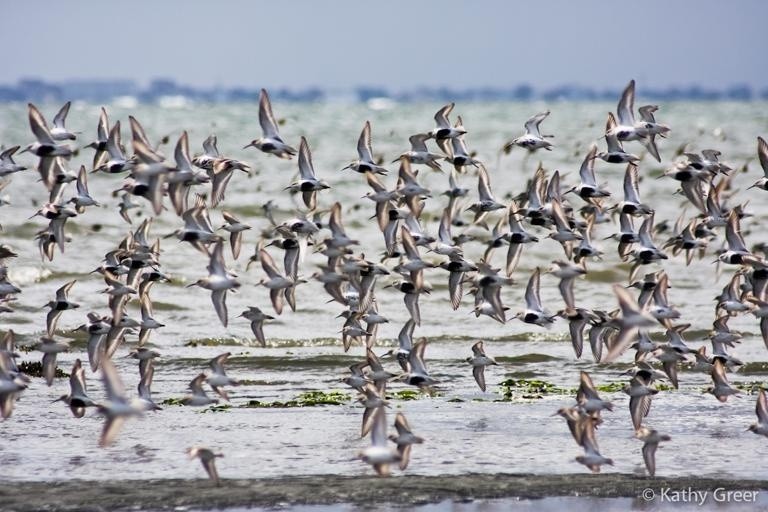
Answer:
[66,165,100,212]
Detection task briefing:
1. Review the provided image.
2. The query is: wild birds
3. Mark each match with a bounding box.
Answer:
[116,183,169,214]
[395,226,436,290]
[184,446,224,488]
[511,207,575,214]
[744,387,767,440]
[673,186,707,197]
[387,412,423,472]
[245,221,319,315]
[701,358,742,405]
[52,394,99,418]
[203,353,244,403]
[745,136,768,192]
[649,310,679,319]
[654,345,688,390]
[439,170,469,213]
[83,105,126,173]
[428,102,468,159]
[71,311,112,374]
[456,261,517,322]
[89,363,163,451]
[596,78,648,145]
[127,294,166,351]
[49,101,81,142]
[562,145,612,212]
[103,295,138,367]
[701,149,734,177]
[468,301,511,324]
[495,203,539,278]
[702,314,742,359]
[184,238,241,328]
[235,306,276,348]
[606,328,618,352]
[335,309,363,326]
[368,202,409,254]
[338,327,373,353]
[503,110,556,154]
[163,192,227,257]
[317,202,360,249]
[169,130,212,217]
[629,425,673,481]
[24,337,76,389]
[637,105,672,163]
[621,371,658,431]
[663,323,691,354]
[117,190,142,223]
[50,357,99,419]
[711,282,730,318]
[283,136,333,212]
[40,279,82,338]
[217,209,252,260]
[1,329,30,421]
[36,231,72,263]
[624,271,673,308]
[17,103,79,194]
[549,407,604,443]
[675,151,719,176]
[591,307,621,364]
[313,248,354,265]
[311,269,348,304]
[543,260,587,309]
[629,329,661,363]
[354,386,393,440]
[741,275,768,349]
[29,173,79,254]
[466,285,484,300]
[507,267,557,330]
[86,119,131,169]
[601,214,641,263]
[435,253,479,310]
[516,216,556,231]
[127,115,179,187]
[356,258,391,311]
[544,198,584,262]
[391,338,441,393]
[717,272,750,313]
[709,204,768,270]
[393,132,445,177]
[550,308,601,358]
[574,371,614,430]
[598,284,667,370]
[607,161,654,221]
[362,172,402,232]
[586,111,641,165]
[176,375,218,412]
[426,210,464,260]
[656,144,703,212]
[0,145,27,174]
[384,270,431,328]
[339,362,378,396]
[537,169,573,215]
[464,341,501,394]
[715,165,743,210]
[339,121,390,180]
[380,318,422,371]
[124,350,163,408]
[242,88,299,160]
[567,424,618,477]
[0,176,22,316]
[194,135,250,208]
[453,116,469,157]
[621,218,669,286]
[464,163,508,224]
[87,216,172,302]
[664,214,708,268]
